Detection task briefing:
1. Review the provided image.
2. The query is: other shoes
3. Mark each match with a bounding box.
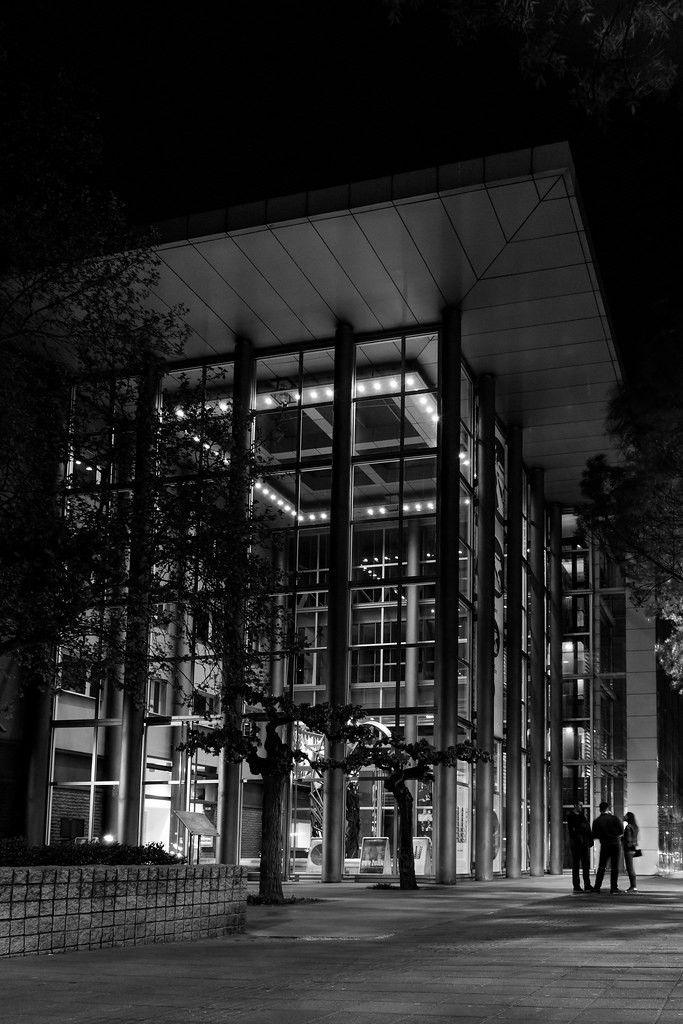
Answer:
[624,888,638,893]
[610,889,627,894]
[573,887,583,892]
[584,885,593,891]
[590,888,600,893]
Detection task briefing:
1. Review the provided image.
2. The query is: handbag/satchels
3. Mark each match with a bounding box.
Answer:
[633,849,642,857]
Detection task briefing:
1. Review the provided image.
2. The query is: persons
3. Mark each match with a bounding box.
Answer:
[568,800,639,893]
